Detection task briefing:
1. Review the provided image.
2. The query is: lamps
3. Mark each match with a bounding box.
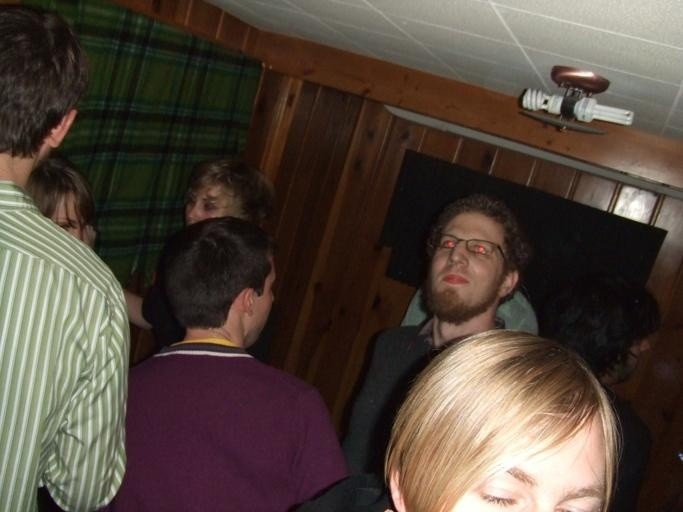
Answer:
[521,62,634,128]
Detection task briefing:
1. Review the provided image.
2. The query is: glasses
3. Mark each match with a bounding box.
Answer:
[432,234,507,262]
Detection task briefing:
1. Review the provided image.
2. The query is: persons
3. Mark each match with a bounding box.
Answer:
[123,151,277,362]
[105,217,355,510]
[25,154,98,253]
[305,194,534,511]
[0,5,132,510]
[537,278,661,512]
[382,327,623,512]
